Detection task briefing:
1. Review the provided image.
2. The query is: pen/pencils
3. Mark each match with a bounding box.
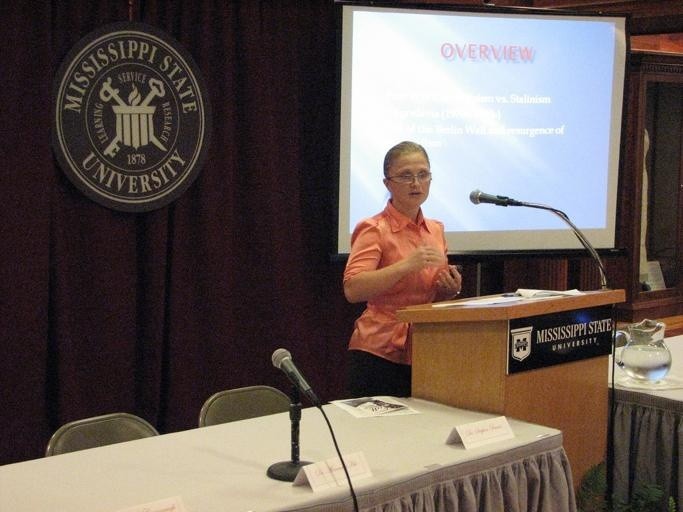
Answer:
[502,293,522,297]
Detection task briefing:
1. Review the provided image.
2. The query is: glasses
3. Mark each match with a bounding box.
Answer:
[388,171,431,182]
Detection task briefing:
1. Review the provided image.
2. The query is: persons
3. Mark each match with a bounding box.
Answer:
[342,142,462,398]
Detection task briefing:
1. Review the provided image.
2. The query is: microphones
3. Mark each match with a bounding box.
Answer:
[271,348,321,409]
[470,191,523,206]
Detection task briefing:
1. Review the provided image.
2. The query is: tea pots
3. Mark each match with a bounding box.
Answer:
[614,318,672,383]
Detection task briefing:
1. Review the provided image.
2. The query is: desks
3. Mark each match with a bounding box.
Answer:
[608,334,683,511]
[0,398,577,512]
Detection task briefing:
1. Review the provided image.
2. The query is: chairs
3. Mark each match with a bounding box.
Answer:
[199,384,293,428]
[46,411,161,457]
